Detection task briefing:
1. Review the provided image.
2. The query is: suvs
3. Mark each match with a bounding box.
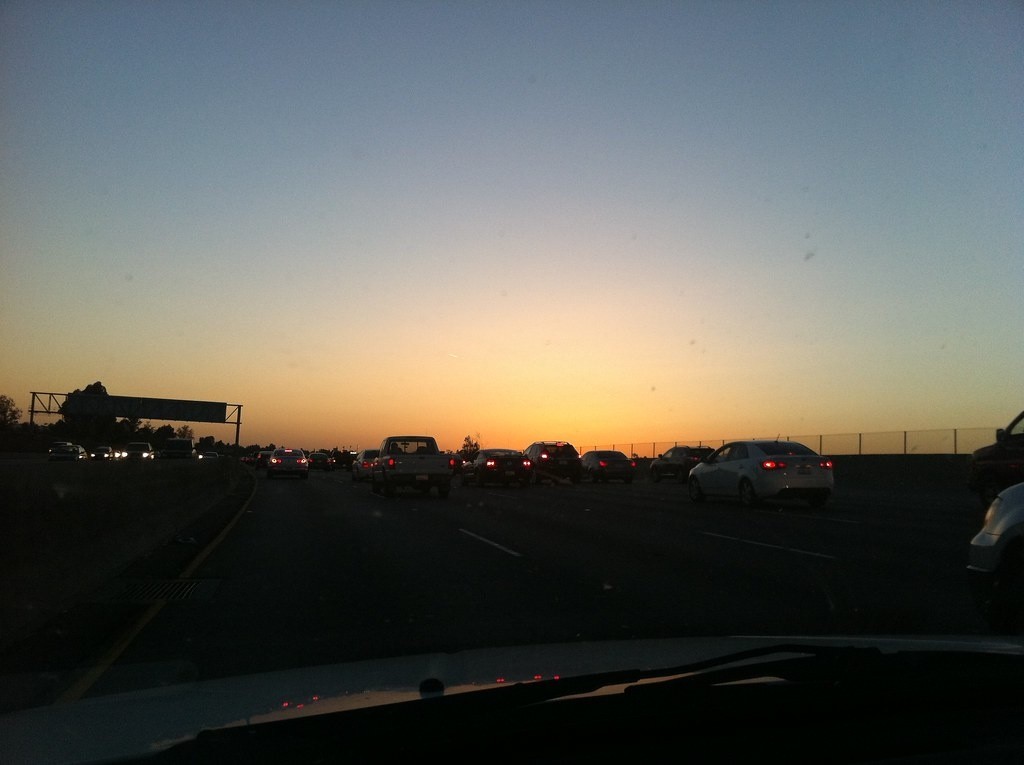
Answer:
[521,440,583,486]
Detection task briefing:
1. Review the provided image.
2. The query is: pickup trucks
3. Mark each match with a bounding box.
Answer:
[369,435,456,497]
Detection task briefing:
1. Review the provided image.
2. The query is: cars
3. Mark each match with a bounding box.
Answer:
[579,450,636,483]
[650,445,718,483]
[687,440,833,511]
[352,449,381,481]
[443,448,537,488]
[267,448,310,481]
[308,452,333,473]
[968,482,1023,636]
[964,409,1024,500]
[43,438,272,476]
